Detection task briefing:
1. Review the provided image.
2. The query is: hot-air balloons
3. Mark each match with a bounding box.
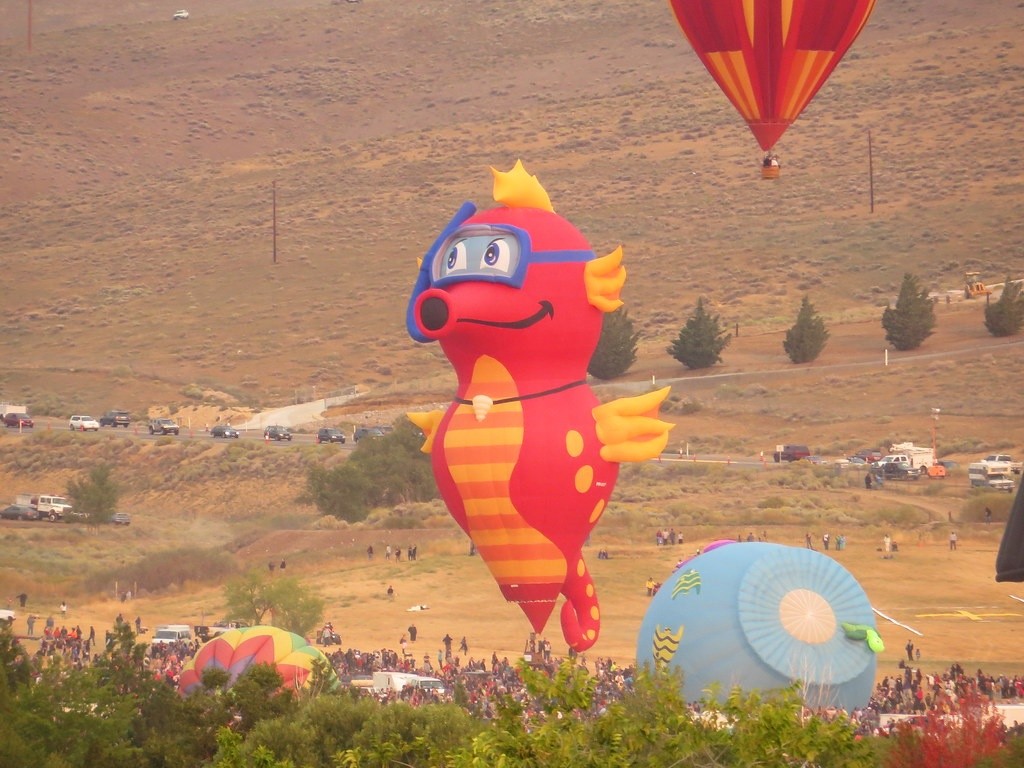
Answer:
[636,537,885,735]
[406,157,679,668]
[176,625,344,727]
[667,0,877,180]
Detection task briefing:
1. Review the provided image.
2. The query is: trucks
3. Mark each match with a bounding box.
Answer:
[17,493,72,522]
[372,671,445,699]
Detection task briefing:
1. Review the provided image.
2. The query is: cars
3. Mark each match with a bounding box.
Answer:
[211,425,239,439]
[69,415,100,432]
[4,412,35,428]
[111,513,131,527]
[0,504,41,522]
[353,425,393,444]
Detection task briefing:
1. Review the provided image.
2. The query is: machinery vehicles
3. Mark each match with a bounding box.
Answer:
[963,271,992,300]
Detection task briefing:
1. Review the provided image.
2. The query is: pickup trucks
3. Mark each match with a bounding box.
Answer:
[969,454,1024,493]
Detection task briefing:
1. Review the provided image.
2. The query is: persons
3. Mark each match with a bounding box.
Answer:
[386,544,417,563]
[815,640,1024,740]
[807,533,846,551]
[764,156,778,166]
[120,591,131,604]
[678,560,682,565]
[865,474,882,490]
[367,546,372,559]
[60,601,67,616]
[657,528,684,546]
[17,591,26,608]
[738,532,768,543]
[10,613,201,697]
[950,532,956,550]
[388,587,393,597]
[316,623,636,733]
[599,550,608,558]
[985,507,992,525]
[268,558,286,573]
[884,534,897,552]
[647,577,660,597]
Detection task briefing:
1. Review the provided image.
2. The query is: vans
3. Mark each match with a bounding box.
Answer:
[151,624,194,648]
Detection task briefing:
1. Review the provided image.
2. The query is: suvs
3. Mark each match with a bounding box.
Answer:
[148,417,180,436]
[774,444,810,464]
[317,426,346,444]
[99,409,131,428]
[264,425,293,441]
[803,441,961,481]
